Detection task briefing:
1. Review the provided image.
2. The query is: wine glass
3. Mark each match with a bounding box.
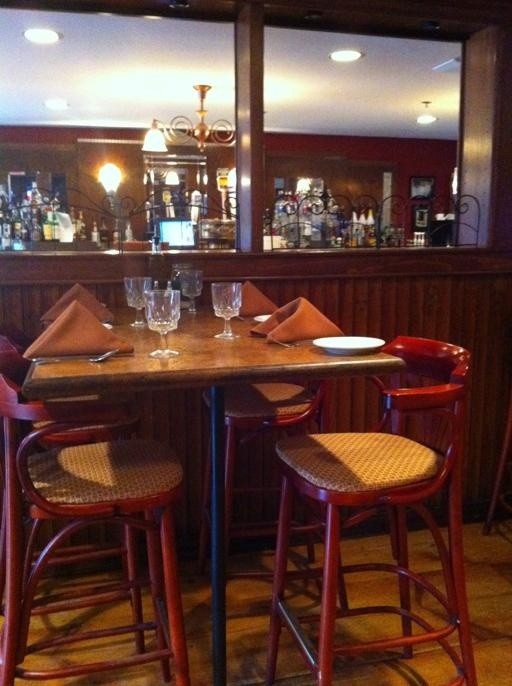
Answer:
[211,282,242,339]
[181,269,204,312]
[124,276,151,328]
[171,263,193,288]
[143,289,180,360]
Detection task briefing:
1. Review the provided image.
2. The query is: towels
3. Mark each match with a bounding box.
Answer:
[22,300,135,359]
[39,283,116,322]
[248,295,344,344]
[231,282,277,319]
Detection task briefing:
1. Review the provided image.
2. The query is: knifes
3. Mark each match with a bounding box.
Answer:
[31,350,136,363]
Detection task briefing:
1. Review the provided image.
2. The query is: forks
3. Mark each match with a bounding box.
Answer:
[264,332,313,349]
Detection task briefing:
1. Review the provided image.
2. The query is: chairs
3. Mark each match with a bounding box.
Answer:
[263,336,478,686]
[194,374,347,609]
[0,371,190,686]
[0,319,147,653]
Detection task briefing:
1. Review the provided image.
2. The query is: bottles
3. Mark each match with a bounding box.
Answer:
[264,188,409,249]
[169,263,193,309]
[0,179,133,251]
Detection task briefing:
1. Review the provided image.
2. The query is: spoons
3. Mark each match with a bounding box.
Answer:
[34,348,121,365]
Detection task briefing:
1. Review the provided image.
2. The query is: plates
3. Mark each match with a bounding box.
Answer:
[313,336,386,355]
[254,314,271,322]
[101,324,114,330]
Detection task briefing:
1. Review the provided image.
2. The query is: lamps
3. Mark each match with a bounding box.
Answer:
[140,84,236,155]
